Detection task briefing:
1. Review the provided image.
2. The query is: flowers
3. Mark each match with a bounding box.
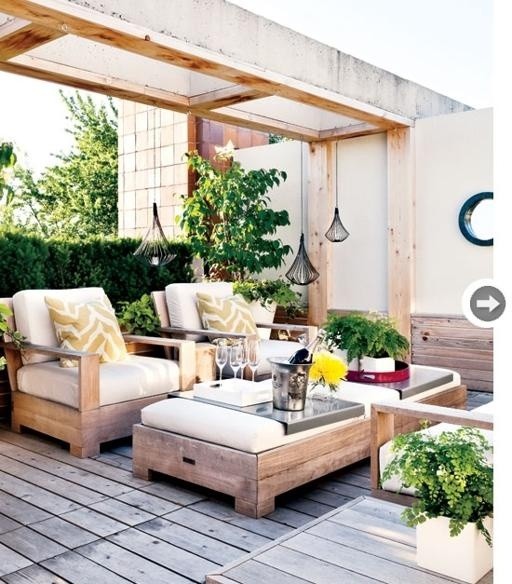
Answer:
[309,357,349,393]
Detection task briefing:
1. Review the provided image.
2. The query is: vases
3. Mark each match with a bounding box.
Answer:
[305,389,316,404]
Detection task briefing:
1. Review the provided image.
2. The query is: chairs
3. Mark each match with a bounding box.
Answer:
[1,286,195,459]
[365,400,493,507]
[149,282,319,382]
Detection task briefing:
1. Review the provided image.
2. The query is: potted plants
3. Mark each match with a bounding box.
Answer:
[174,148,299,340]
[368,422,494,583]
[322,309,408,372]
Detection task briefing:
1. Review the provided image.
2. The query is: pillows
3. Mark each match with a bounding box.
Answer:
[44,295,127,367]
[197,293,261,346]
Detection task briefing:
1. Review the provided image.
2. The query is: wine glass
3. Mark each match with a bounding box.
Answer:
[216,338,261,392]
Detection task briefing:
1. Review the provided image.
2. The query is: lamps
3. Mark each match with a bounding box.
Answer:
[284,131,320,285]
[130,111,178,268]
[325,140,350,244]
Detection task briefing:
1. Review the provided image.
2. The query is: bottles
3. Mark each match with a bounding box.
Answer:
[285,329,327,364]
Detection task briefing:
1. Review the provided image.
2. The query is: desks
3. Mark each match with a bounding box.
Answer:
[205,494,493,583]
[130,355,470,522]
